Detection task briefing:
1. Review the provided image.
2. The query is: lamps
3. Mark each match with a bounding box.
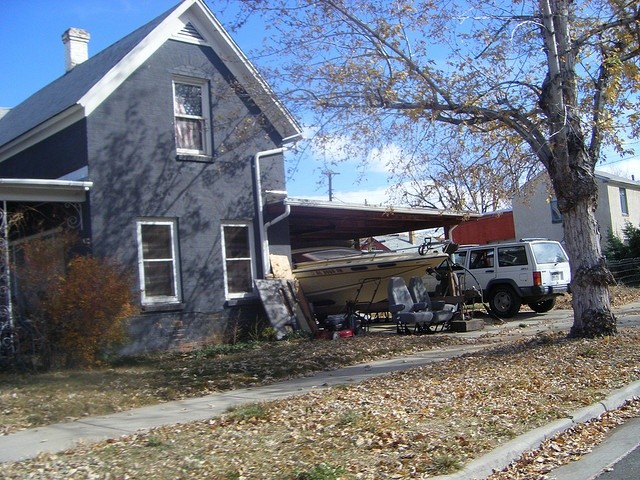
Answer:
[409,277,453,334]
[387,277,433,335]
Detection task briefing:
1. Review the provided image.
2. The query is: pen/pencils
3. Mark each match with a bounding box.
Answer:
[427,238,571,317]
